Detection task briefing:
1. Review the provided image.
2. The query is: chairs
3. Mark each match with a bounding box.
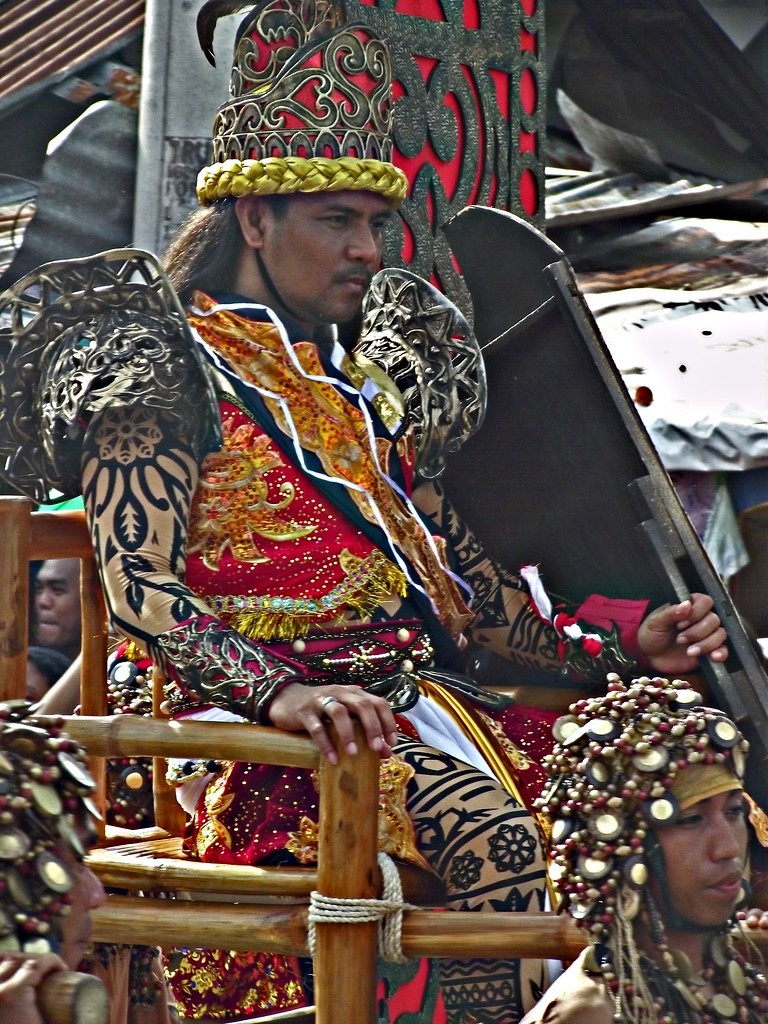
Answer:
[0,495,706,1024]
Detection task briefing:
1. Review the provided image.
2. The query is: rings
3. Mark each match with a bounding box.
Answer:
[322,696,337,709]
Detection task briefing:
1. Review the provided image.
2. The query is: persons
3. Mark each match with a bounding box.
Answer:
[0,0,729,1024]
[25,558,83,703]
[521,672,767,1024]
[0,699,105,1024]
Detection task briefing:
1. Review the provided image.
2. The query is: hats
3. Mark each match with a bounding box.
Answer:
[190,1,410,205]
[0,701,103,956]
[537,674,767,1024]
